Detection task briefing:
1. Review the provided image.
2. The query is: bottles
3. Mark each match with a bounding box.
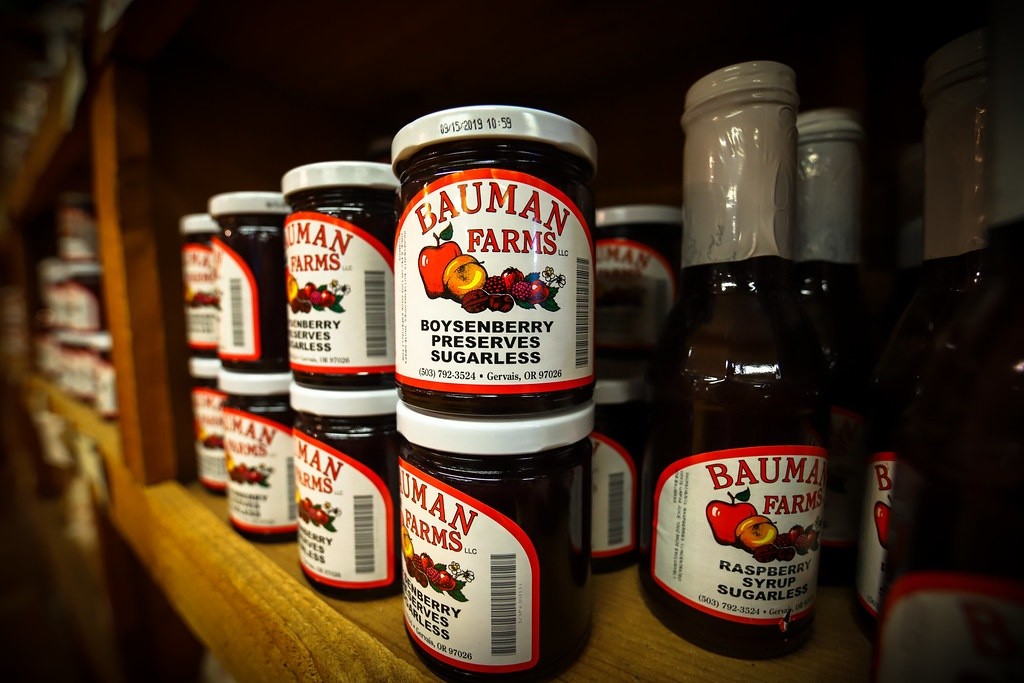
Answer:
[638,32,1024,661]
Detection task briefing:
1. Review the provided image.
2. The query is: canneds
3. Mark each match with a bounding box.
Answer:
[39,105,687,683]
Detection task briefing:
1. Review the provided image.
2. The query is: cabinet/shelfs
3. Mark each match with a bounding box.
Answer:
[0,0,871,683]
